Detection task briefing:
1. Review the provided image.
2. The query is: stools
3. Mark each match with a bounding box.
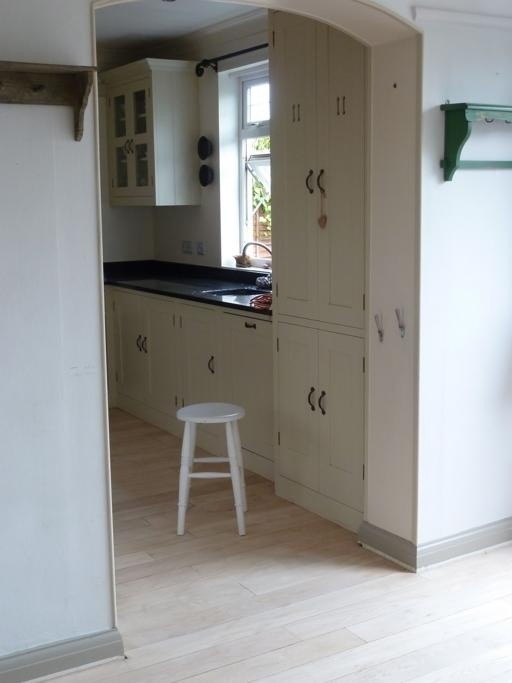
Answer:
[174,402,250,539]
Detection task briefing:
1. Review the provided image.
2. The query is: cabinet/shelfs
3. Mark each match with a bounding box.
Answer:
[222,310,272,465]
[99,56,202,207]
[273,315,367,528]
[270,9,367,334]
[179,299,220,441]
[114,286,176,420]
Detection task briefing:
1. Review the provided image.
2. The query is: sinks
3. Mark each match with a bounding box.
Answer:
[197,284,271,302]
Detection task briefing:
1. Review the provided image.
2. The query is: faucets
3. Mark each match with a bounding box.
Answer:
[242,241,272,259]
